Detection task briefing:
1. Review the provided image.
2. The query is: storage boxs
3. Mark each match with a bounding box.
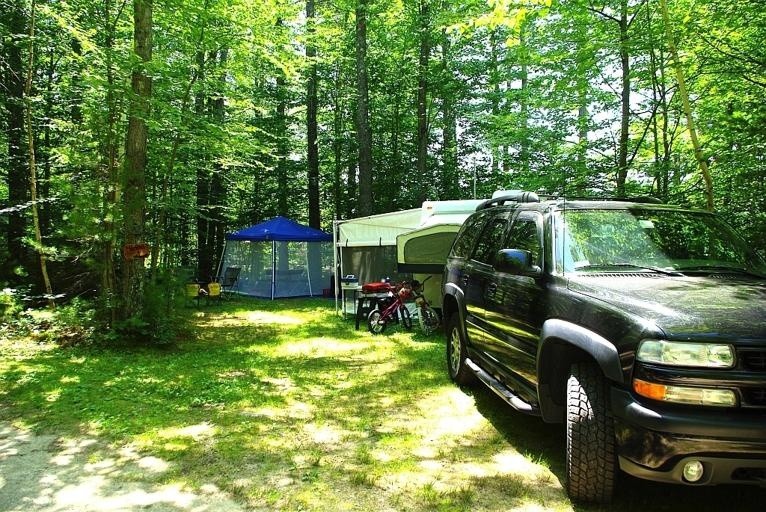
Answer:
[340,274,358,288]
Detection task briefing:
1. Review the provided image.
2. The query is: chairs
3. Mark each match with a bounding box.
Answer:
[182,264,242,308]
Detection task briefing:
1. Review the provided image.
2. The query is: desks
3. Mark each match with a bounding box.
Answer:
[341,286,364,322]
[353,293,398,331]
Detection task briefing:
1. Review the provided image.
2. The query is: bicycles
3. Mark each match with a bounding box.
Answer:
[405,273,440,337]
[367,281,412,335]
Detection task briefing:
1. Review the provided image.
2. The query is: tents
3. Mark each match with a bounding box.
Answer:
[333,200,490,321]
[214,216,335,300]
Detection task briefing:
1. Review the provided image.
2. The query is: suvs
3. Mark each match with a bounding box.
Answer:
[437,187,766,505]
